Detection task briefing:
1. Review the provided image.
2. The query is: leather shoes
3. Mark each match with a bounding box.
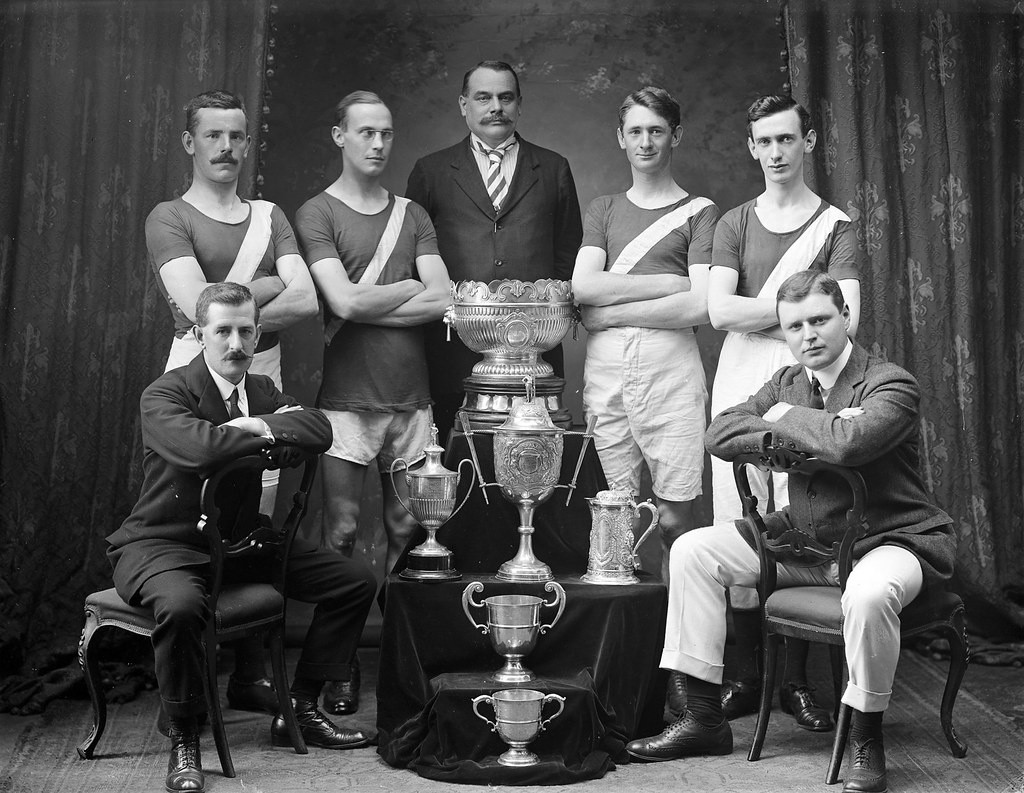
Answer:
[782,685,832,731]
[719,681,765,721]
[667,673,688,715]
[157,701,208,739]
[624,709,733,762]
[165,740,205,792]
[843,739,888,793]
[322,652,362,716]
[227,675,297,716]
[270,704,368,749]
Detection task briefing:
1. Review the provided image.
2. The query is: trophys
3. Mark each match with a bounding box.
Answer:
[460,375,598,585]
[389,423,476,583]
[472,690,566,766]
[445,277,575,419]
[462,580,566,684]
[581,491,660,588]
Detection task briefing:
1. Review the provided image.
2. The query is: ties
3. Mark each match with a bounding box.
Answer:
[226,389,244,421]
[808,376,825,411]
[470,136,516,215]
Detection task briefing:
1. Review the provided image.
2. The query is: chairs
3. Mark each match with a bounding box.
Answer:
[733,445,969,786]
[77,444,318,778]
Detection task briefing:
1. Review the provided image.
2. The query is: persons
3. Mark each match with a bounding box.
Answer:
[707,95,860,732]
[626,270,959,793]
[145,90,320,737]
[572,87,721,720]
[295,90,456,714]
[105,282,369,793]
[405,62,584,473]
